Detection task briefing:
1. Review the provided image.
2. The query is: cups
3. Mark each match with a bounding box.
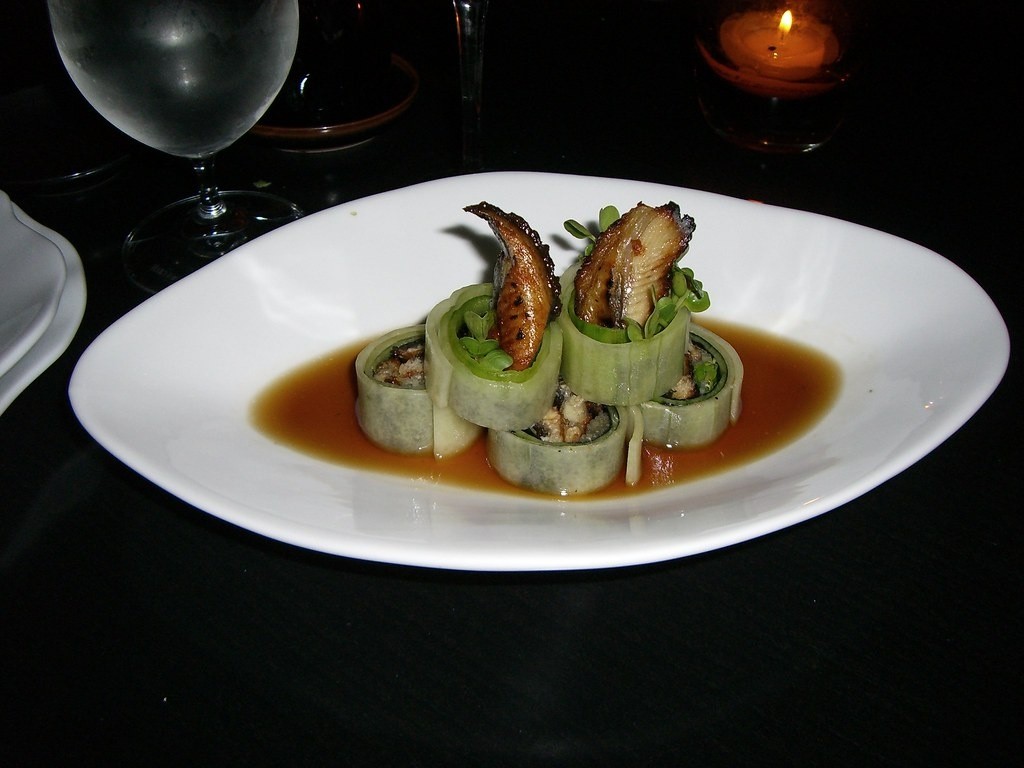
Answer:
[448,0,490,178]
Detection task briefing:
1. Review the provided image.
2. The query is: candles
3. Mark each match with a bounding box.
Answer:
[743,8,825,69]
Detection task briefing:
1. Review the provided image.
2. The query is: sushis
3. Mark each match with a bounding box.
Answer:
[352,279,744,496]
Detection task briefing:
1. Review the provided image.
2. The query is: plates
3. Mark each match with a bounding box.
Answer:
[0,186,87,420]
[68,171,1011,570]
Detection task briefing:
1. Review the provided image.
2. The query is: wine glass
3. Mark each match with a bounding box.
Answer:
[46,0,304,297]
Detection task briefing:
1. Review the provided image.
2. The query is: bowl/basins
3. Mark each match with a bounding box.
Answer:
[248,46,421,154]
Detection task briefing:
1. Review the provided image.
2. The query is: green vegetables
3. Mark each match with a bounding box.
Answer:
[452,205,711,368]
